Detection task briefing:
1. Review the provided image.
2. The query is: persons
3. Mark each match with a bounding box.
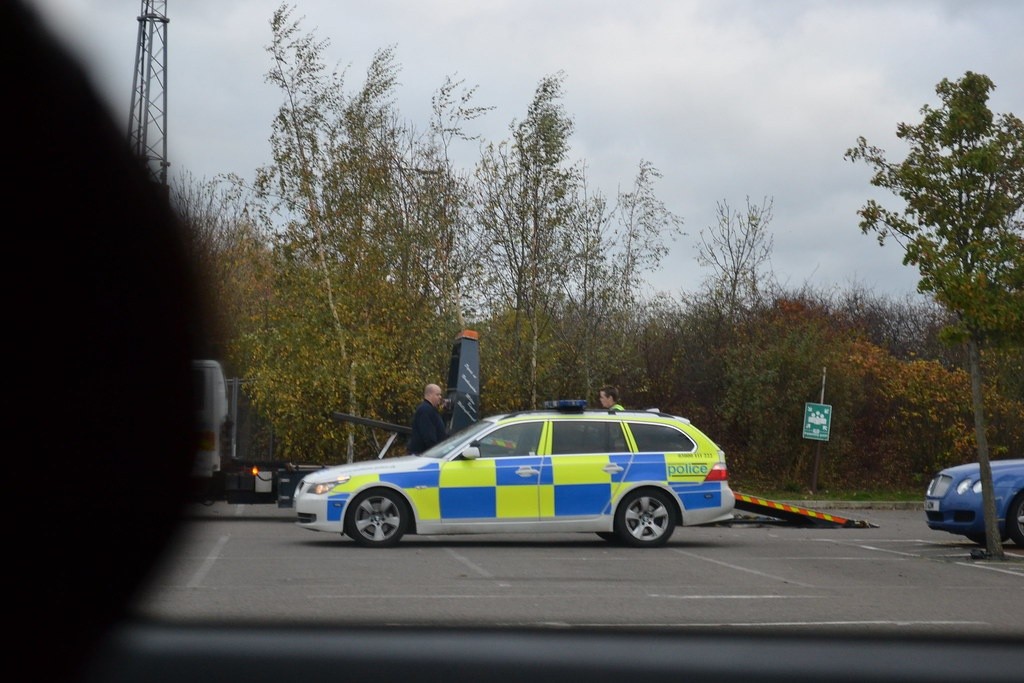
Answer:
[600,386,625,411]
[412,383,447,454]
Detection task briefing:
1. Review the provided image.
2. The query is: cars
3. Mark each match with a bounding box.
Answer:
[923,457,1024,552]
[192,360,230,483]
[292,400,735,549]
